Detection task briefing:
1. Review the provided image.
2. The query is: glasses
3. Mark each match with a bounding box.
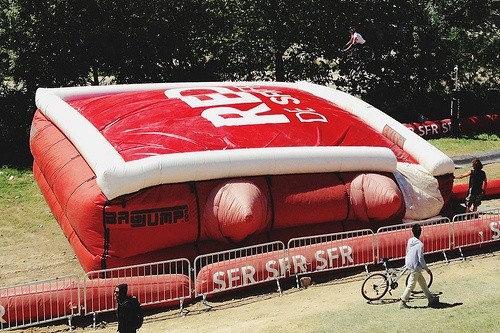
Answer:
[114,290,120,294]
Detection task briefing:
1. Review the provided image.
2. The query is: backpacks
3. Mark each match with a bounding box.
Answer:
[120,297,144,329]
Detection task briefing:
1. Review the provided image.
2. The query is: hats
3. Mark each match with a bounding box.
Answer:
[116,284,127,295]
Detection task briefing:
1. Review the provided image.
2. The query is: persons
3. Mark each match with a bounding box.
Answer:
[114,284,143,333]
[452,159,487,220]
[398,223,439,310]
[342,27,366,54]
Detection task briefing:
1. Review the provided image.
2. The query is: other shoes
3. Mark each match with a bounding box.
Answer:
[399,304,411,309]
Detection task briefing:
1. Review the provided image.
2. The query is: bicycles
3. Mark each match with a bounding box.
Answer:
[361,256,433,301]
